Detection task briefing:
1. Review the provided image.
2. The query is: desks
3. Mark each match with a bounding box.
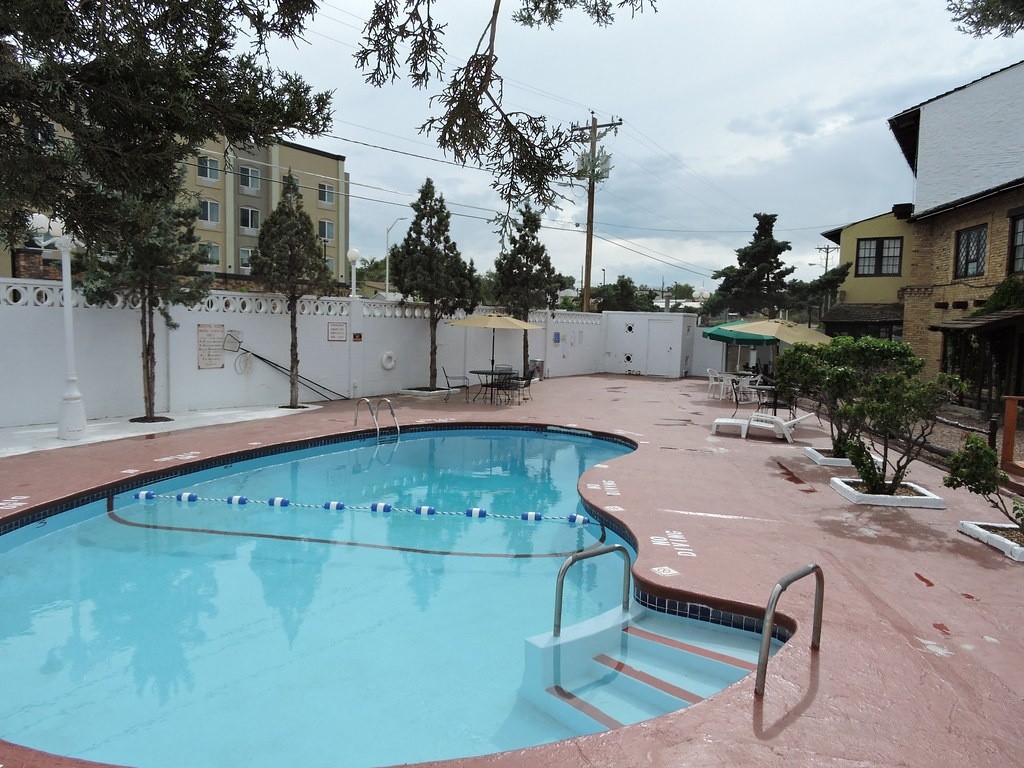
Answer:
[748,386,799,416]
[469,370,516,403]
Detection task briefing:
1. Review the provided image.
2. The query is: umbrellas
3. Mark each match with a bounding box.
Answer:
[444,313,545,404]
[702,320,780,372]
[719,318,833,416]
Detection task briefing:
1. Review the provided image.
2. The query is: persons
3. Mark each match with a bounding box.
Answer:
[746,362,760,375]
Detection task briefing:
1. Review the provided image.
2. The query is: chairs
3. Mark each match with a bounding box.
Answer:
[442,366,470,403]
[706,362,797,420]
[711,413,815,444]
[492,364,535,406]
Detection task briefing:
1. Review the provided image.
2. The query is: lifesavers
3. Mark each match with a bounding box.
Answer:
[382,350,396,369]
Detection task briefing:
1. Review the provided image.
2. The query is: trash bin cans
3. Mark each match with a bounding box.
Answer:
[529,359,545,381]
